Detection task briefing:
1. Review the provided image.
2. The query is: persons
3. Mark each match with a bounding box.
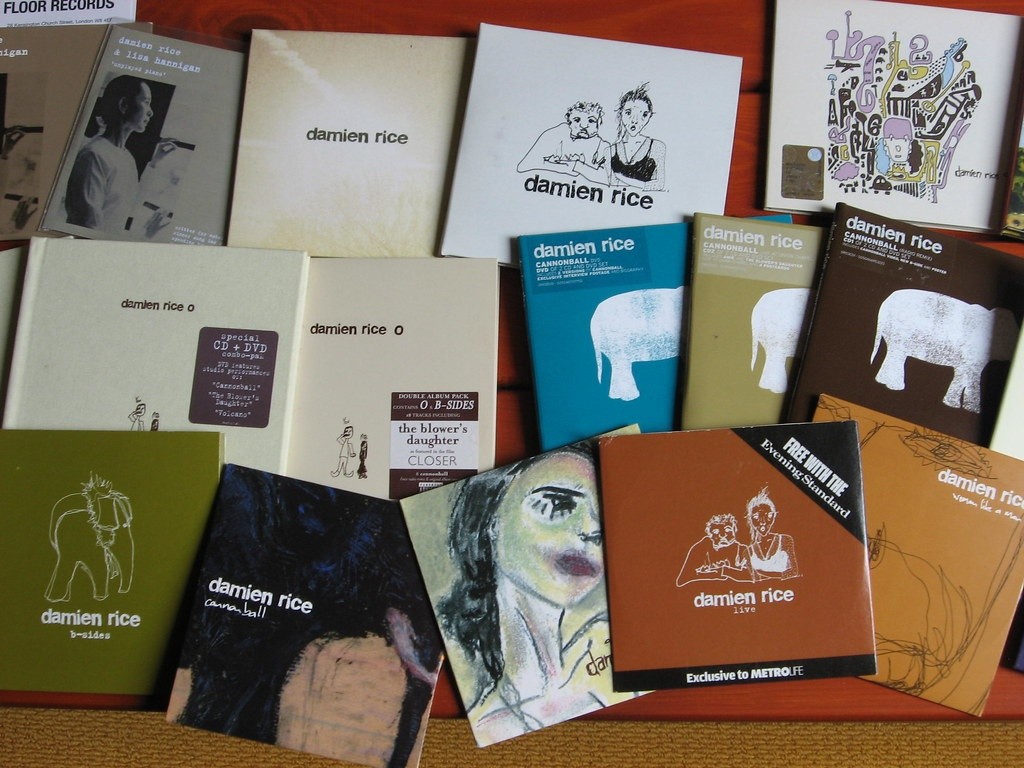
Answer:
[64,75,179,239]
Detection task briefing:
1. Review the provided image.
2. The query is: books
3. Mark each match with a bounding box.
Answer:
[0,0,1024,768]
